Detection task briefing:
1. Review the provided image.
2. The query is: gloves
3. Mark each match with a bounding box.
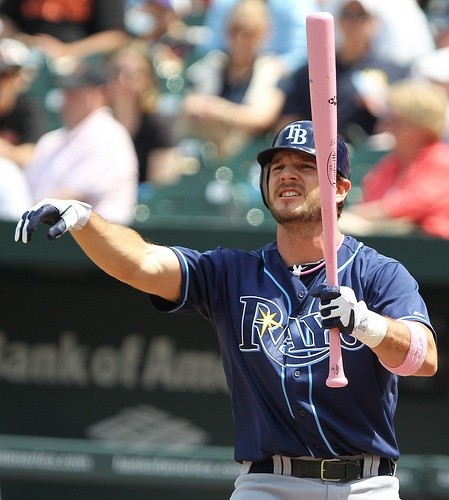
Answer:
[310,284,388,349]
[14,198,91,244]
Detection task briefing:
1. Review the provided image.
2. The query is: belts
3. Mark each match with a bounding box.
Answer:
[249,457,394,482]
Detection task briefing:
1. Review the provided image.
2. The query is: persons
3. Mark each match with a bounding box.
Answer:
[0,0,449,239]
[16,120,440,500]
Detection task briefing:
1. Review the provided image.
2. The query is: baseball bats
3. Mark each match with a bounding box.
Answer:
[306,12,348,388]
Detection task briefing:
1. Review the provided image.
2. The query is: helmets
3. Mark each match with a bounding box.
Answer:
[257,121,351,179]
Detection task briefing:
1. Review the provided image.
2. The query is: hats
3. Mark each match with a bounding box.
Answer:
[384,79,447,138]
[344,0,398,40]
[51,62,108,87]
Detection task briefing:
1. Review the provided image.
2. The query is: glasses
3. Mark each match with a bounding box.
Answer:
[339,10,365,23]
